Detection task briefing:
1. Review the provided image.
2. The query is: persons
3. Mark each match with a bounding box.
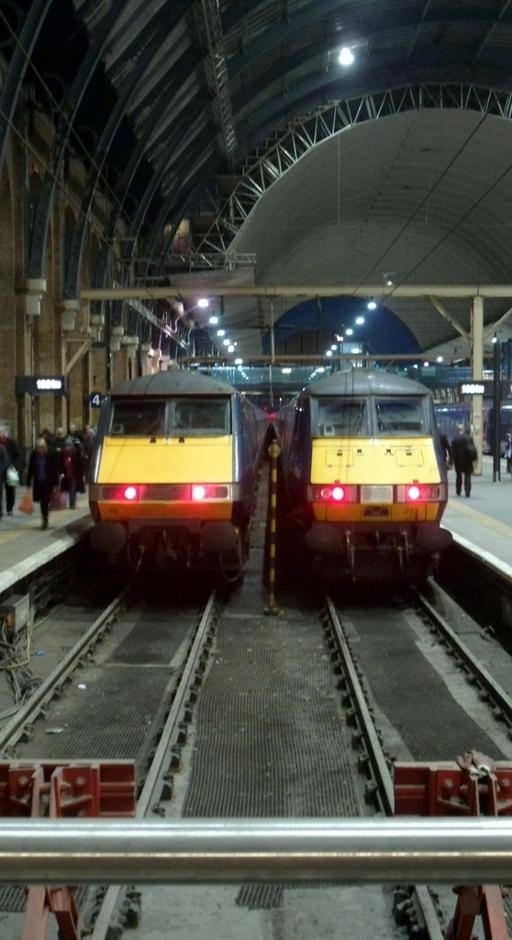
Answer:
[449,423,478,500]
[503,432,511,475]
[0,423,99,532]
[438,429,453,477]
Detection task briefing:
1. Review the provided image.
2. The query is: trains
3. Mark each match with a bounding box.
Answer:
[88,369,270,585]
[268,367,453,599]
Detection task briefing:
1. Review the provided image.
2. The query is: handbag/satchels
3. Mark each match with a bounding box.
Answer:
[6,466,20,487]
[18,496,33,515]
[50,488,67,511]
